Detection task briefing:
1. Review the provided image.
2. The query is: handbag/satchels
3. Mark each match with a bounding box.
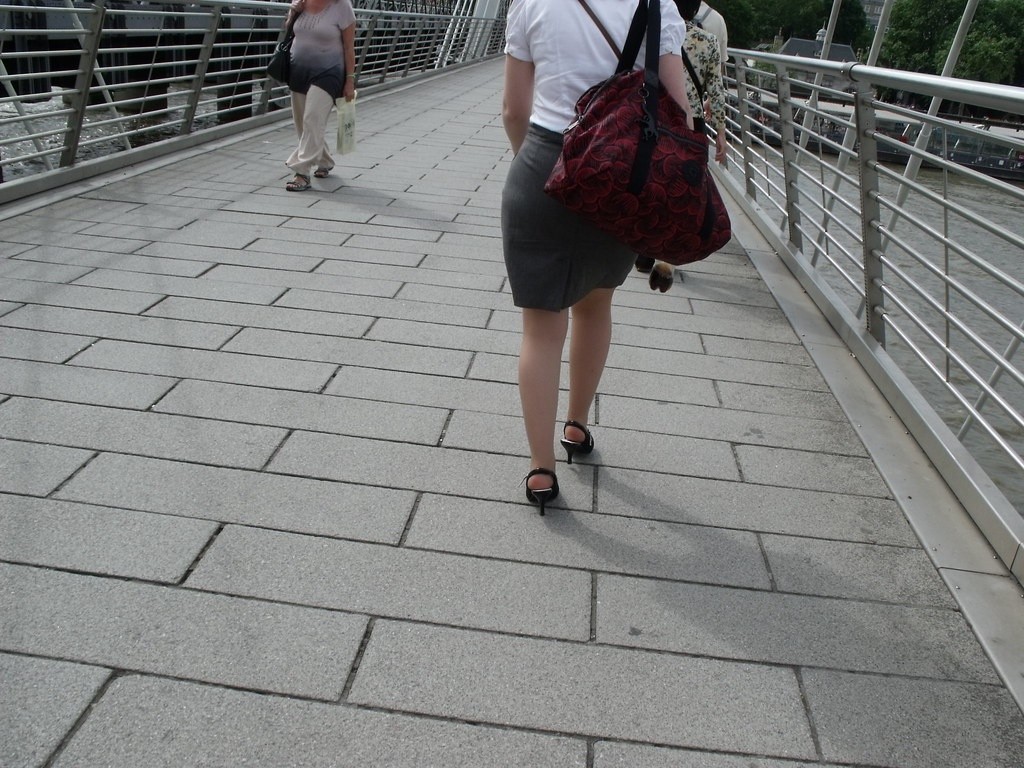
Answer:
[544,71,732,266]
[335,90,357,155]
[267,42,289,82]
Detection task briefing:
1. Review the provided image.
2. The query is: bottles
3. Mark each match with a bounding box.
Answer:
[649,260,674,293]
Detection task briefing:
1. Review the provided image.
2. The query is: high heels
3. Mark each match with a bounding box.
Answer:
[560,420,594,464]
[518,468,559,516]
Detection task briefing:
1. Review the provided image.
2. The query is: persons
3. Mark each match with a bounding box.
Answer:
[692,0,729,122]
[635,0,728,273]
[501,0,696,516]
[283,0,356,192]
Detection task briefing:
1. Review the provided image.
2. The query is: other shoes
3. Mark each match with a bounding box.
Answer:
[636,255,655,271]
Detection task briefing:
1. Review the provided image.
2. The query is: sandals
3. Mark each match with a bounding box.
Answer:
[313,169,332,178]
[286,173,312,191]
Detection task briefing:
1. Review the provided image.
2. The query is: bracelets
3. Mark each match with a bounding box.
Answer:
[346,75,355,78]
